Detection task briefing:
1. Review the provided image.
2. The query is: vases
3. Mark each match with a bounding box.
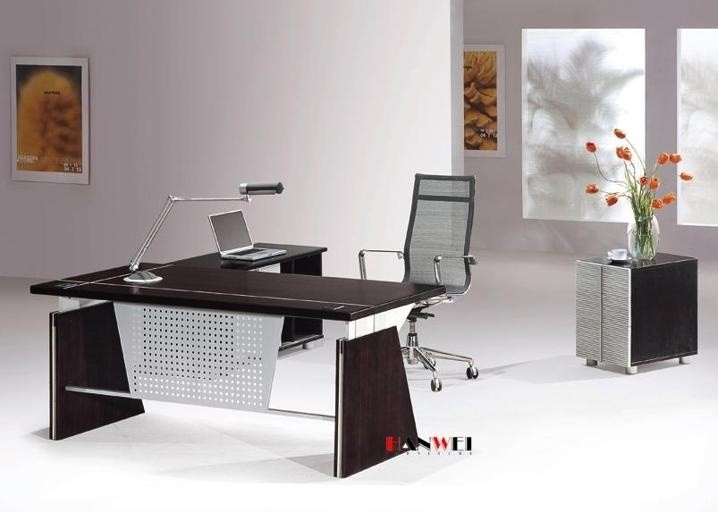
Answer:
[625,212,661,262]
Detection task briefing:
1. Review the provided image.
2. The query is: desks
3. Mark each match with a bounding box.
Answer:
[29,240,446,482]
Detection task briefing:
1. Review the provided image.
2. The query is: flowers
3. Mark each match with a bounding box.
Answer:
[584,126,699,263]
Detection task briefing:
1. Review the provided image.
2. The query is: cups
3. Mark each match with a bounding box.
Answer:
[607,248,628,261]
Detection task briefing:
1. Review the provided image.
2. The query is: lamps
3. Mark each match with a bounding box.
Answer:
[124,177,286,283]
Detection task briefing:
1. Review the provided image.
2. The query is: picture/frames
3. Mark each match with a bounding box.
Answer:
[6,54,92,187]
[461,40,509,162]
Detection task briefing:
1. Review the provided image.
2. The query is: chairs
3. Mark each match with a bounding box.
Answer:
[355,172,482,392]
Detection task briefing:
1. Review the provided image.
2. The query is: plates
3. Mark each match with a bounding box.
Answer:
[604,256,633,262]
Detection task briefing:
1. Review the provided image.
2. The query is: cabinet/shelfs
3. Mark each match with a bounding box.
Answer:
[574,252,703,375]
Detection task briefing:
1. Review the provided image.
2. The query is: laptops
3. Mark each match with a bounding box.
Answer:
[208,209,287,261]
